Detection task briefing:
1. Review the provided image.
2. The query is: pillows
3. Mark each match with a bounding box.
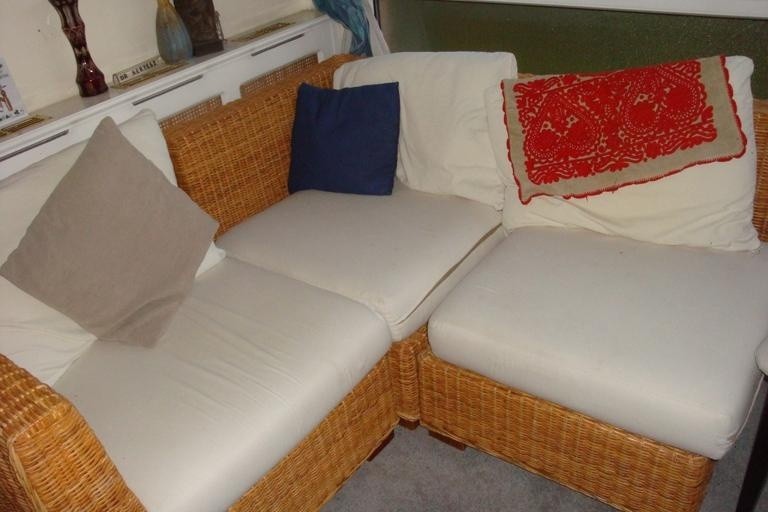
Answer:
[332,48,518,210]
[0,115,222,348]
[287,81,400,197]
[484,54,760,250]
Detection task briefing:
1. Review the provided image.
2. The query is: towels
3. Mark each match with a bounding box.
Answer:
[500,59,746,208]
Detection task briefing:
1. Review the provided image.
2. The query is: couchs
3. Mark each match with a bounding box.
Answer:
[0,54,768,512]
